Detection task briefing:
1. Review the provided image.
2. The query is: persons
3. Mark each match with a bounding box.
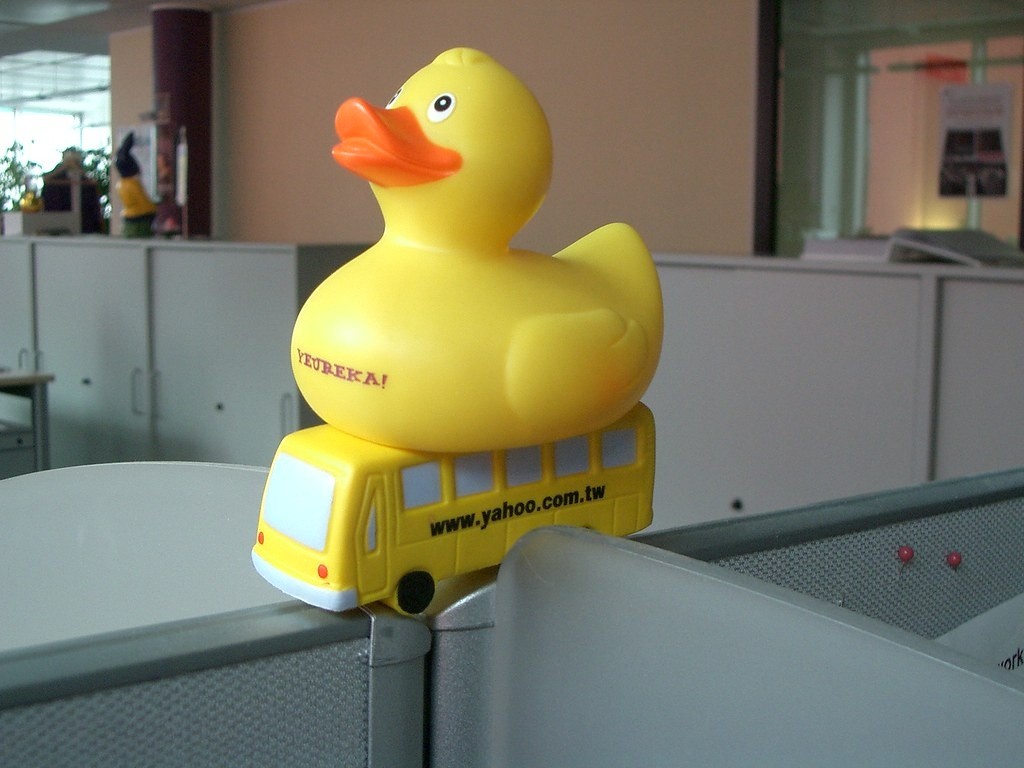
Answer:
[44,149,85,179]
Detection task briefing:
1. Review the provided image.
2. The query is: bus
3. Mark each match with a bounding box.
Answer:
[250,399,657,617]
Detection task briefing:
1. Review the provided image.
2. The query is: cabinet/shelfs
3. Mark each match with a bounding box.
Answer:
[0,233,379,479]
